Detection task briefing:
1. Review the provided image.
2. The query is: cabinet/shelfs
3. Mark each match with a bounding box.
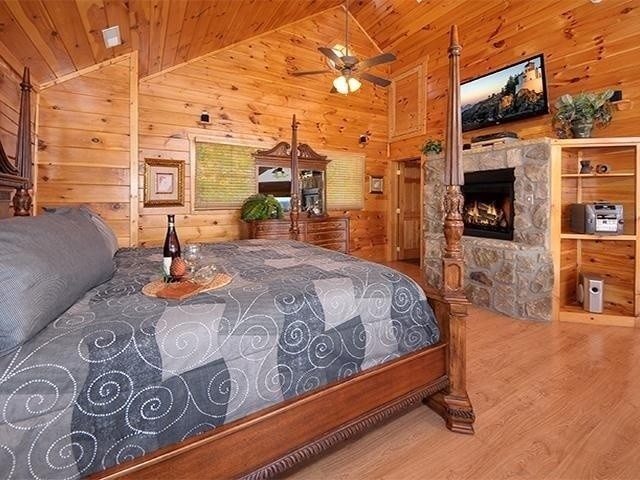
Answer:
[236,213,353,255]
[543,140,639,326]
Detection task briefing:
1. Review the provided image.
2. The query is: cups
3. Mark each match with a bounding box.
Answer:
[192,263,219,287]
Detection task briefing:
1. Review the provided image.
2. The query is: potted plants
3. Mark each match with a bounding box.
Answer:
[419,138,442,156]
[547,85,620,139]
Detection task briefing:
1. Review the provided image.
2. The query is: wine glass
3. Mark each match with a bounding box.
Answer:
[184,244,202,281]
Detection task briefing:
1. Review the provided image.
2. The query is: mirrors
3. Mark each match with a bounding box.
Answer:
[249,142,333,217]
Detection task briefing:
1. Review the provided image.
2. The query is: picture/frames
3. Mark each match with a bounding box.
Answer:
[368,175,384,195]
[142,158,186,208]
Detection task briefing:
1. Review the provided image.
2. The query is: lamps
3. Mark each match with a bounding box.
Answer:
[331,71,364,96]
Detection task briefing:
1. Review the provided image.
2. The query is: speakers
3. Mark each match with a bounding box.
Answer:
[569,201,586,235]
[597,164,610,174]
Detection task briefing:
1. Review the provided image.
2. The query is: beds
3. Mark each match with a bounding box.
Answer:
[1,22,476,480]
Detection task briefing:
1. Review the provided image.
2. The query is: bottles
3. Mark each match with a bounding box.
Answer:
[161,213,181,279]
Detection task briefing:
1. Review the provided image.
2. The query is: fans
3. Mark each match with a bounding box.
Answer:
[286,0,401,99]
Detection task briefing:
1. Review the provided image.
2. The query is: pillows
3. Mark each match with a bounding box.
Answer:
[1,210,118,356]
[44,203,120,261]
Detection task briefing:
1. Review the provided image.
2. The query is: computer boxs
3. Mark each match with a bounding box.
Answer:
[579,272,603,314]
